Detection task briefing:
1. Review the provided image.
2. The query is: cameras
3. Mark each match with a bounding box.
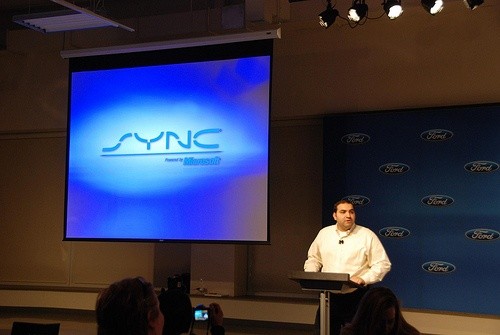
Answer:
[193,307,210,321]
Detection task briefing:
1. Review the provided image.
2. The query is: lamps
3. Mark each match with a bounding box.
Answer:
[420,0,444,16]
[318,0,338,29]
[348,0,368,23]
[466,0,484,10]
[381,0,404,20]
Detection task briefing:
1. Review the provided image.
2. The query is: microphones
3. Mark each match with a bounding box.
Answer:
[340,240,344,244]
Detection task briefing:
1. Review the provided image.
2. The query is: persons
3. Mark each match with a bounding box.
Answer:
[340,287,421,335]
[303,199,392,335]
[203,312,208,319]
[94,276,226,335]
